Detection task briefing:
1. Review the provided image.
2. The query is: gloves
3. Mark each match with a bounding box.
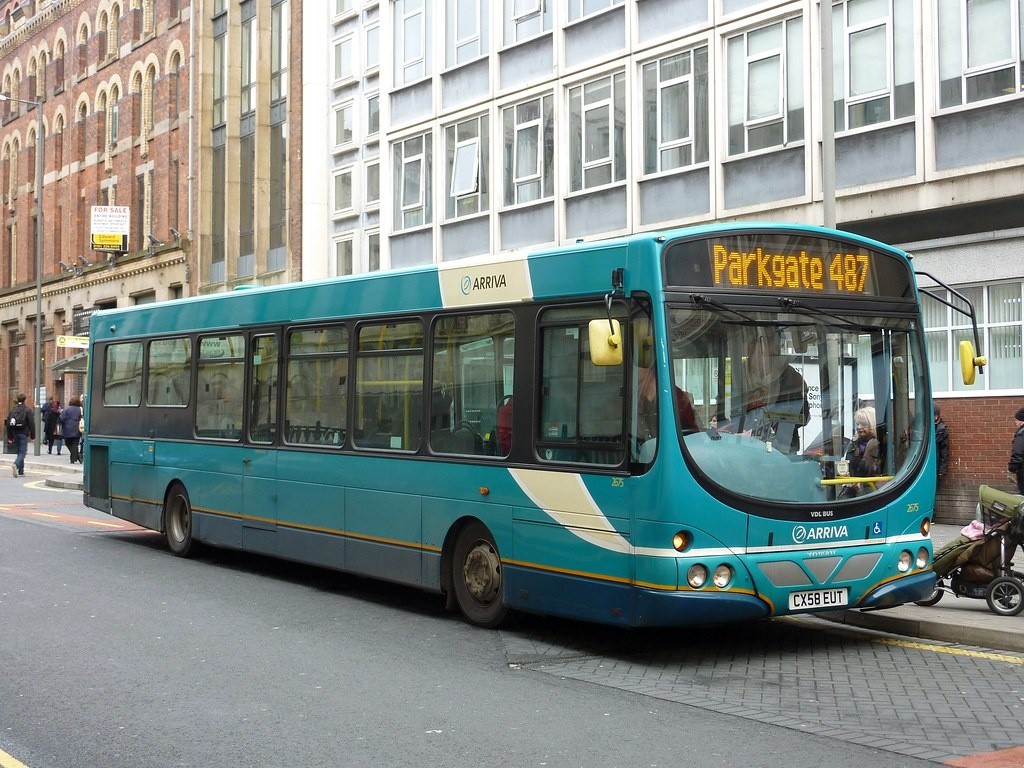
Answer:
[1007,470,1017,487]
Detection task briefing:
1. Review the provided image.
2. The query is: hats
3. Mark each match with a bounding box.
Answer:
[1015,408,1024,421]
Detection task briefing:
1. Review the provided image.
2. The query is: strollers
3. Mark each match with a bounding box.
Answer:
[914,483,1024,617]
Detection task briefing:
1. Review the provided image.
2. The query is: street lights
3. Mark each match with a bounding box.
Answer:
[0,95,42,454]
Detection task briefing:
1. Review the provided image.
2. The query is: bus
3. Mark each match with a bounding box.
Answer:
[81,222,988,628]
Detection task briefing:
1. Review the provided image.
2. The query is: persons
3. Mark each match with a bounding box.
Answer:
[739,364,811,452]
[837,406,882,501]
[1008,407,1024,496]
[930,404,949,525]
[40,396,85,465]
[178,357,211,405]
[6,392,36,478]
[638,366,700,437]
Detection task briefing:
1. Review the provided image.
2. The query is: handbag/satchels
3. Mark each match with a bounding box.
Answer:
[53,424,63,435]
[78,407,85,433]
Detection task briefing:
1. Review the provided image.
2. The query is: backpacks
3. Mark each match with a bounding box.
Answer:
[9,407,31,431]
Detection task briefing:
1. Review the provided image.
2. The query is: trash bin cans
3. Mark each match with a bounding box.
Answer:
[3,419,20,455]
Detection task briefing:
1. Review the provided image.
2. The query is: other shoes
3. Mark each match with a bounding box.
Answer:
[77,454,82,464]
[12,464,18,478]
[57,453,61,454]
[19,472,24,475]
[47,451,51,454]
